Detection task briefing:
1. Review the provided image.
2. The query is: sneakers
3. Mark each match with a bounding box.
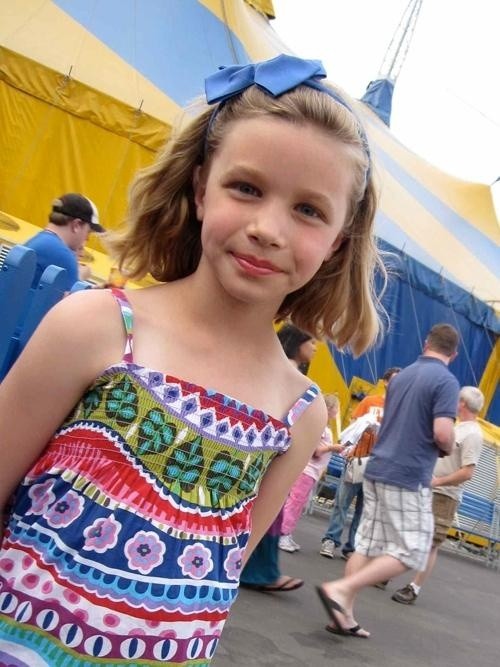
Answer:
[289,533,300,551]
[318,539,336,560]
[277,536,296,555]
[338,546,355,557]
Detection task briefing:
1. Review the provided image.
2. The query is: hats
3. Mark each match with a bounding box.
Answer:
[50,193,107,236]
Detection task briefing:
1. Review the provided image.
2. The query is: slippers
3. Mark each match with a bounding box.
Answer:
[323,624,373,641]
[313,584,352,636]
[255,574,305,592]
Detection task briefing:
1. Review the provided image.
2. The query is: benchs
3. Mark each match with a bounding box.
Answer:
[303,452,500,565]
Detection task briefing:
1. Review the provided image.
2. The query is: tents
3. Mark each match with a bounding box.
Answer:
[0,1,500,451]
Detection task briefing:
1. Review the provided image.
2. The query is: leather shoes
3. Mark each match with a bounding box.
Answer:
[391,585,418,605]
[376,579,390,587]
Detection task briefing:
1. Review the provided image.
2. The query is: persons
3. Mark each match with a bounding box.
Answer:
[240,324,318,593]
[1,192,113,379]
[314,322,460,636]
[279,394,343,553]
[320,366,402,561]
[372,386,485,605]
[1,54,386,667]
